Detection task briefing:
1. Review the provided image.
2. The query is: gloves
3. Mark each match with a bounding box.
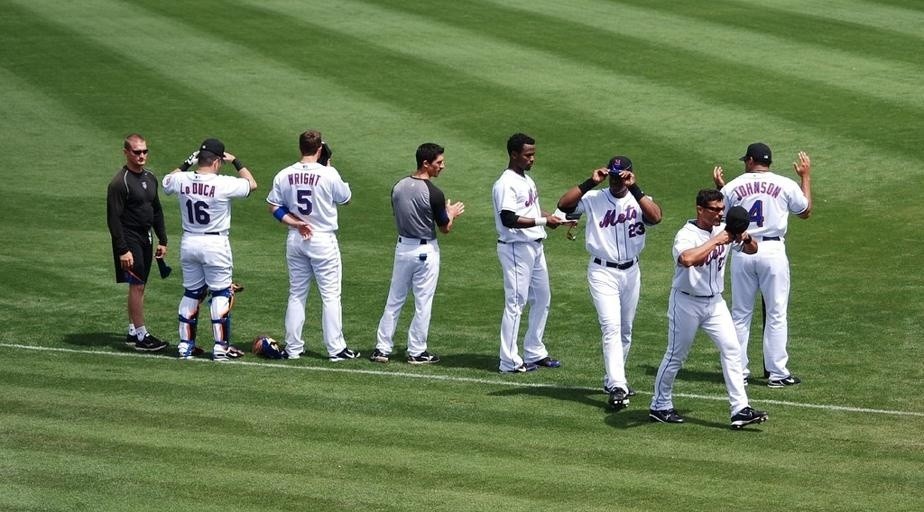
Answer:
[183,151,199,167]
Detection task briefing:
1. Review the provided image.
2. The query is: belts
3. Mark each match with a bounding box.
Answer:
[593,258,638,270]
[398,237,426,244]
[763,237,779,240]
[497,238,541,244]
[682,291,713,298]
[183,229,219,235]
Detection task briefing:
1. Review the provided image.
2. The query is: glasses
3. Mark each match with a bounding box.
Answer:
[566,224,585,240]
[132,149,148,154]
[704,206,725,212]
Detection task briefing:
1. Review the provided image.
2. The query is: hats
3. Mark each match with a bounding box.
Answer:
[724,206,749,234]
[739,143,771,162]
[317,142,331,166]
[605,156,632,175]
[199,139,224,157]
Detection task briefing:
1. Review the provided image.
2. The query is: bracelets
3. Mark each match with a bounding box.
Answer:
[625,183,646,204]
[533,216,549,229]
[741,235,753,245]
[273,206,289,223]
[230,158,245,171]
[178,162,191,171]
[576,177,596,193]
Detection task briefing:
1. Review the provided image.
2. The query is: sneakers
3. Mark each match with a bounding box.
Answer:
[369,349,389,362]
[124,333,136,344]
[407,351,439,364]
[534,357,560,368]
[133,332,168,352]
[498,363,536,373]
[212,343,243,361]
[603,375,801,429]
[177,342,203,359]
[287,345,305,360]
[328,347,360,363]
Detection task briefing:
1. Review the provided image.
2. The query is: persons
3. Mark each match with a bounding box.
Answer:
[557,154,663,410]
[105,133,174,354]
[267,130,363,362]
[648,188,770,429]
[371,141,466,367]
[160,140,261,361]
[490,131,563,374]
[710,140,813,390]
